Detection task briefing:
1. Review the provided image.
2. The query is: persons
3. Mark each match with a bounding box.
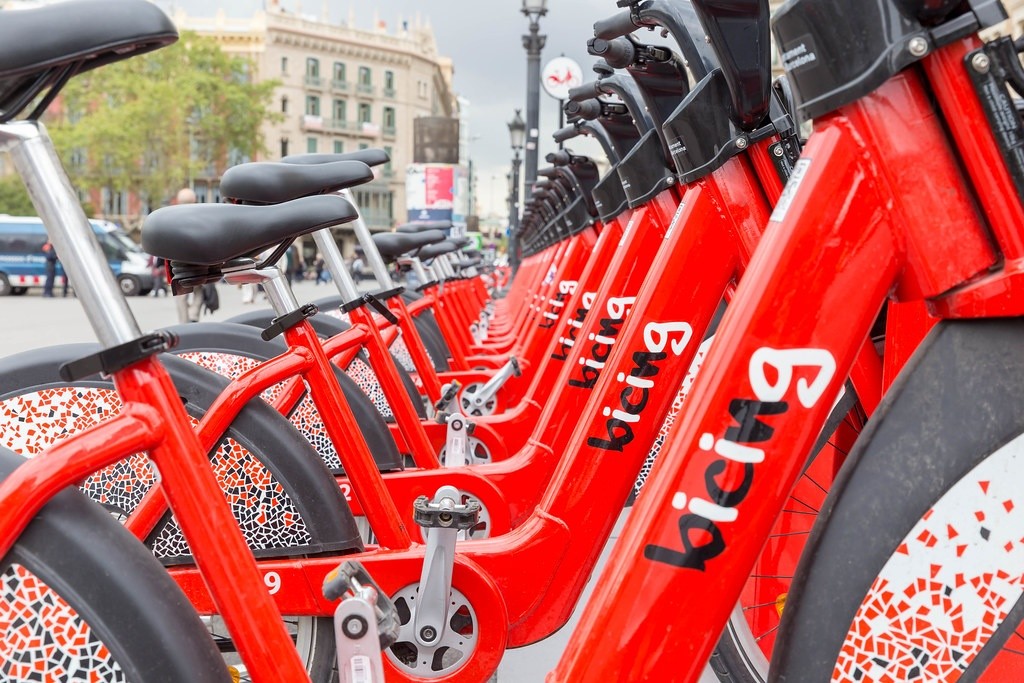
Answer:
[41,188,402,324]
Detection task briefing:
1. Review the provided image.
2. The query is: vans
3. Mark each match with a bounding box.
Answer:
[0,214,155,297]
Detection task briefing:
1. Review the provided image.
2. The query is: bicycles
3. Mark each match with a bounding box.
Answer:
[0,0,1024,683]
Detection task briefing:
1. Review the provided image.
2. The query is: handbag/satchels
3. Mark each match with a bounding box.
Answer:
[204,284,219,310]
